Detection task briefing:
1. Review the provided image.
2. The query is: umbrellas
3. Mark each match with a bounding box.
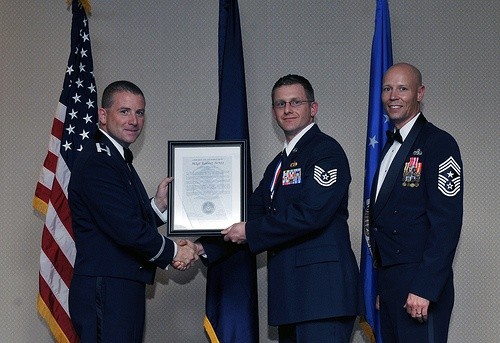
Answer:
[203,0,260,343]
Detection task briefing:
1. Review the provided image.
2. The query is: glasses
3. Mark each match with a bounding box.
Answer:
[273,100,314,108]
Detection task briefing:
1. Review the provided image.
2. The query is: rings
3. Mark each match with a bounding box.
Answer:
[417,312,422,315]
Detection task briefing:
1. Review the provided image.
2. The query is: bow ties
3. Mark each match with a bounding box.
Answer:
[386,128,403,144]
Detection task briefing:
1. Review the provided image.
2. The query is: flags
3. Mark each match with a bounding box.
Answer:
[361,0,394,343]
[32,1,98,343]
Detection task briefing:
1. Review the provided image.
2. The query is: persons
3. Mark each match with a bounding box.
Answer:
[172,74,362,343]
[370,63,465,343]
[67,80,198,343]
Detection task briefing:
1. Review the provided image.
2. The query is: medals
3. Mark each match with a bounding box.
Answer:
[402,174,420,188]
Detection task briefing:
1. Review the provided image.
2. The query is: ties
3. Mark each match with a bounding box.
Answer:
[123,147,134,172]
[280,148,286,168]
